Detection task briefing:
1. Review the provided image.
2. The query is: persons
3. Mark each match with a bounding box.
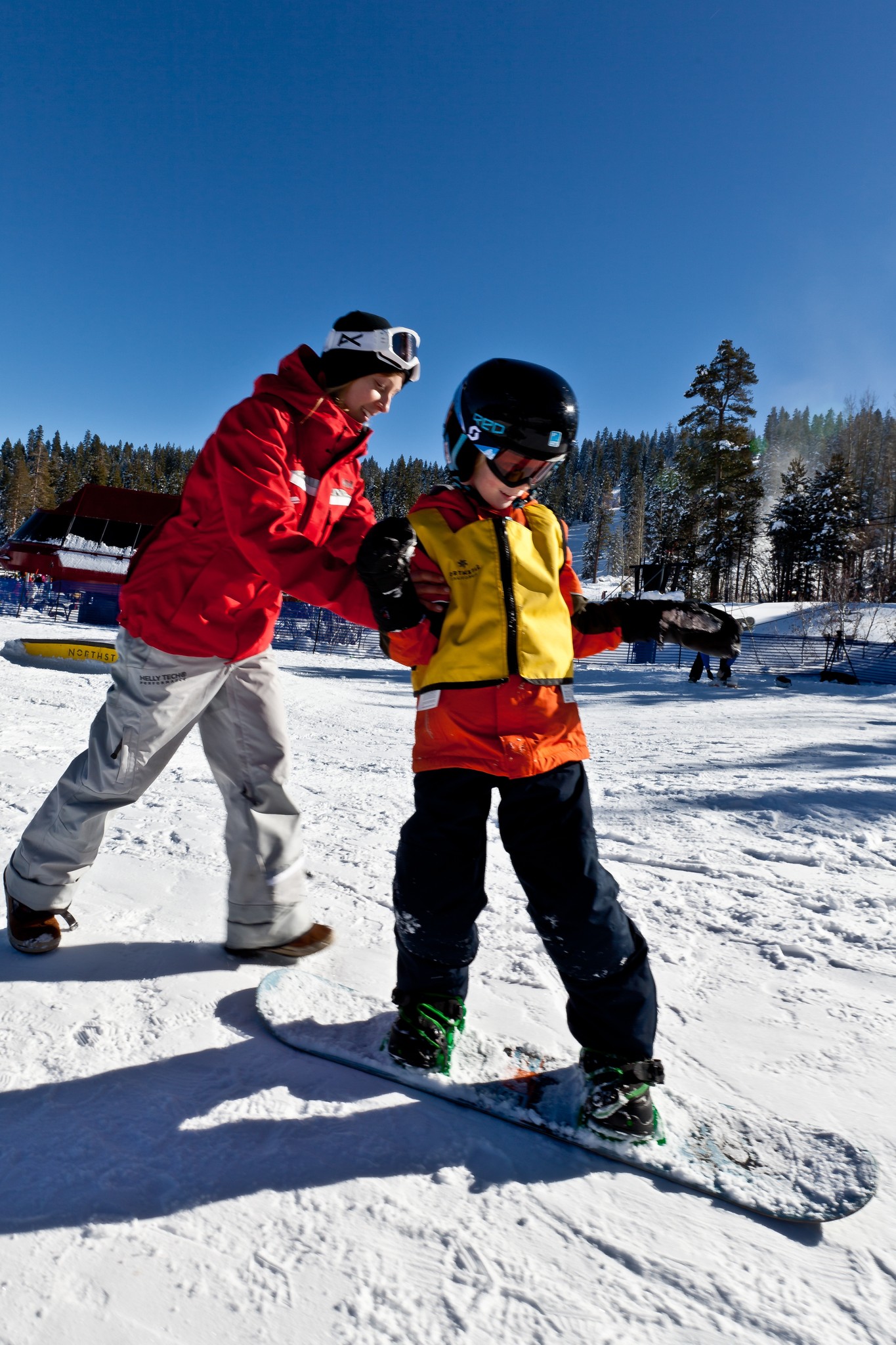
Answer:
[3,309,421,961]
[355,357,739,1146]
[5,574,46,608]
[688,652,737,685]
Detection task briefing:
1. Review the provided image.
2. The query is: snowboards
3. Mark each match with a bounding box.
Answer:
[256,968,880,1224]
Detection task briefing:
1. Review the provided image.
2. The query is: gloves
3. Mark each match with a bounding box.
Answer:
[706,670,714,680]
[621,598,744,661]
[355,518,425,632]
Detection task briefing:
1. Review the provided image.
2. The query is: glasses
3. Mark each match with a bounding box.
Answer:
[376,327,420,382]
[483,446,567,489]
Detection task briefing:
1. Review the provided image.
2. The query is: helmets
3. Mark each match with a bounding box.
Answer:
[443,358,580,482]
[775,675,793,687]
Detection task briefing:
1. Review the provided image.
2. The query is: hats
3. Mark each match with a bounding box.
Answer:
[317,310,413,393]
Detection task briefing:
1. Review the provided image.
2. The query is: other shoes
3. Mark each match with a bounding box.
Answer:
[3,866,79,954]
[578,1046,654,1143]
[721,679,731,686]
[688,677,697,683]
[251,924,334,958]
[389,995,463,1070]
